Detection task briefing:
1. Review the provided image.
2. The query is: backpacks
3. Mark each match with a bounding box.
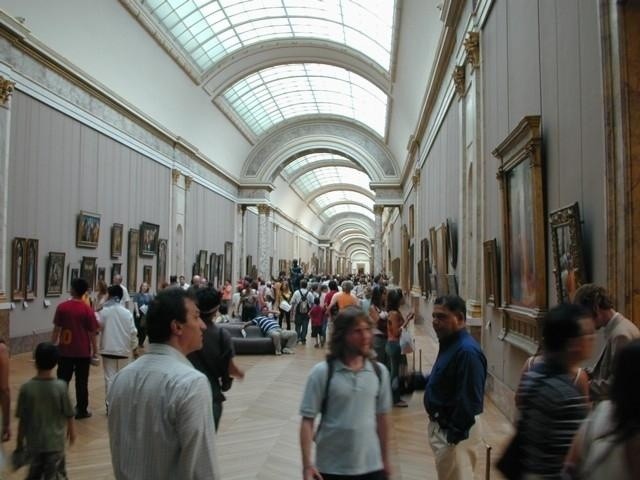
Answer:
[295,288,312,316]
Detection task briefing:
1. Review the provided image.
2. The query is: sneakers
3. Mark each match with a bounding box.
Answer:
[274,347,297,356]
[74,412,93,419]
[394,399,410,408]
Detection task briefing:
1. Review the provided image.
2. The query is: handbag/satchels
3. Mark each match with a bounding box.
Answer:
[329,302,340,316]
[400,326,414,355]
[278,299,293,312]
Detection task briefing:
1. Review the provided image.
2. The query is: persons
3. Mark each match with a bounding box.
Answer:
[51,278,100,419]
[0,338,12,442]
[573,282,640,407]
[514,339,591,405]
[92,260,417,432]
[107,287,221,480]
[15,341,77,480]
[561,337,640,479]
[424,293,488,480]
[299,305,396,480]
[495,304,597,479]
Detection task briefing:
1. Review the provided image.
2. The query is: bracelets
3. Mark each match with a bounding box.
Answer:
[303,464,316,470]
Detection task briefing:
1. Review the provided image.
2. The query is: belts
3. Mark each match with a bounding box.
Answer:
[427,413,439,423]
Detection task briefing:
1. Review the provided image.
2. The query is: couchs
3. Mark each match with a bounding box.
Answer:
[214,320,289,354]
